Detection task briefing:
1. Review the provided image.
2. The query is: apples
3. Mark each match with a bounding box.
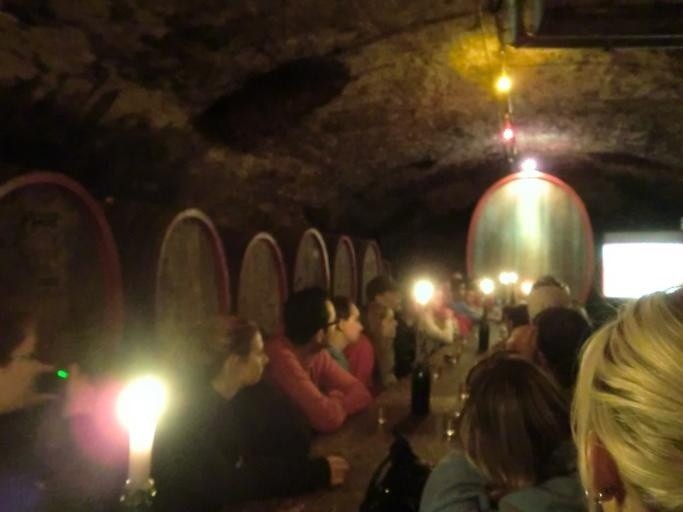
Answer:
[128,425,153,483]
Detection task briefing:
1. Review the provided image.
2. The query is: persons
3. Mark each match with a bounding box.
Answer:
[568,285,682,511]
[261,288,372,435]
[0,286,130,512]
[495,275,615,391]
[402,274,488,355]
[416,352,586,509]
[360,276,415,389]
[149,315,352,508]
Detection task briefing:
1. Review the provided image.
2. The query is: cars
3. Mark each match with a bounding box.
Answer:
[443,409,460,438]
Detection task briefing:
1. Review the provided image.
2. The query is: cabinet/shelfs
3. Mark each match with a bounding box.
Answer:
[411,363,431,417]
[478,304,490,352]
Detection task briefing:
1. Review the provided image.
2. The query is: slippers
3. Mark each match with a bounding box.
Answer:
[359,433,432,512]
